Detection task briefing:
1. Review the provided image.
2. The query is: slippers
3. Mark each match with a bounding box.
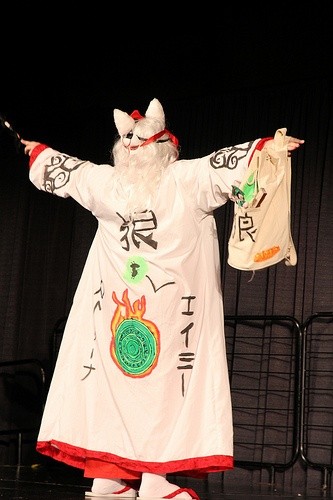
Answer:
[135,486,199,500]
[84,484,137,497]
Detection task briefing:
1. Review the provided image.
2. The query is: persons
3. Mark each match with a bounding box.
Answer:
[20,97,307,500]
